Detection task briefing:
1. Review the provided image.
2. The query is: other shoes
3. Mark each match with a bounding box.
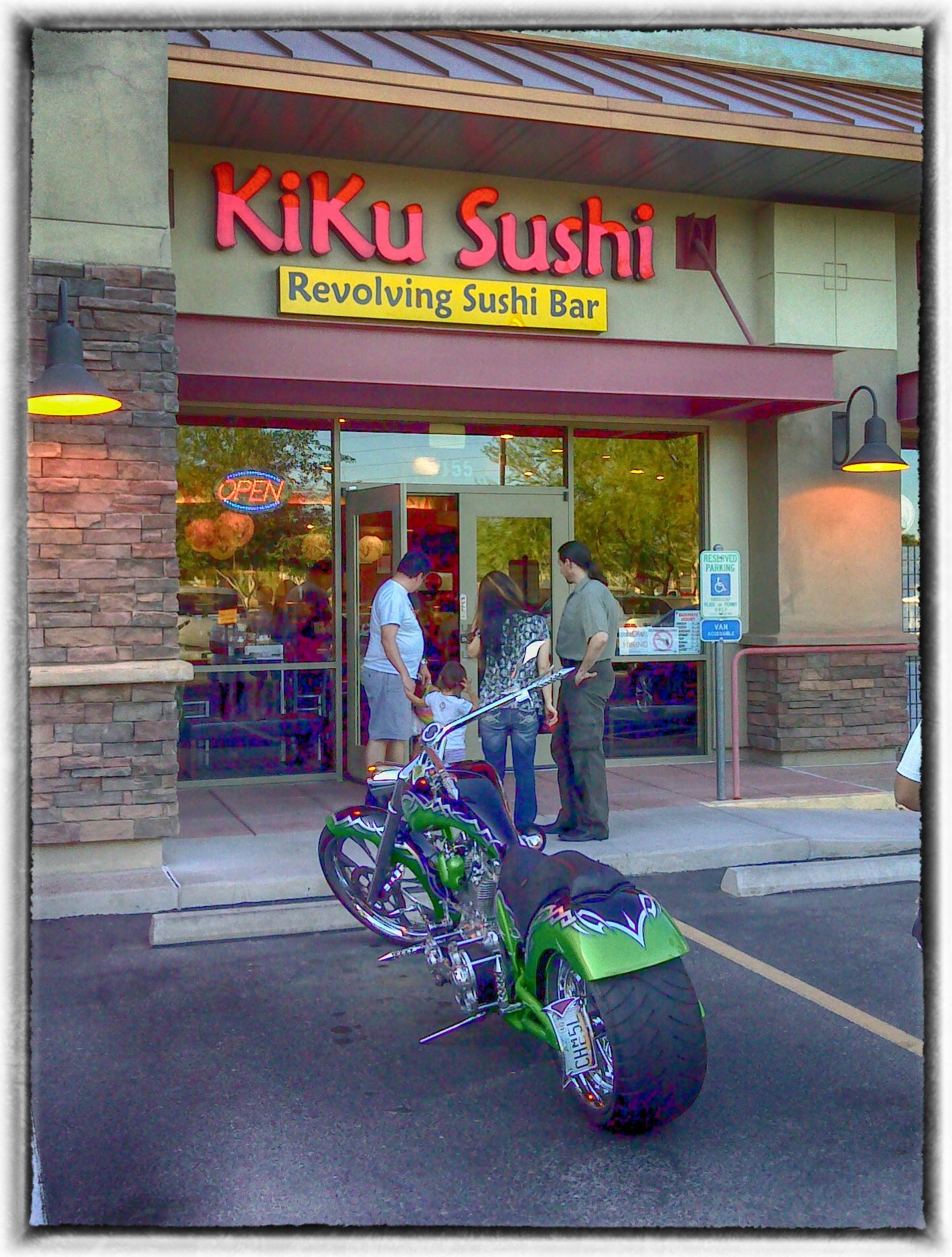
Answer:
[522,829,539,836]
[365,789,392,809]
[539,823,558,833]
[557,830,608,841]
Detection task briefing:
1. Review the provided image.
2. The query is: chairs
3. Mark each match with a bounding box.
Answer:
[182,654,336,766]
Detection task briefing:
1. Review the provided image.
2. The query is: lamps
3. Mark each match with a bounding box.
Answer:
[27,279,123,418]
[830,385,909,474]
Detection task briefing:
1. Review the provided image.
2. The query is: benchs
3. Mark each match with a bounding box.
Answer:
[180,713,330,766]
[606,704,698,757]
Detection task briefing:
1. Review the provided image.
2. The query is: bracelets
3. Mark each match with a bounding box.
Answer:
[420,658,427,665]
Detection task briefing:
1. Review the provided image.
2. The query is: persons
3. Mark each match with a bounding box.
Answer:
[538,540,619,842]
[362,549,432,768]
[893,718,923,948]
[403,660,477,763]
[465,570,558,833]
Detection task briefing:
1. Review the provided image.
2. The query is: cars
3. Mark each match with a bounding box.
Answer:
[175,587,250,651]
[539,592,676,636]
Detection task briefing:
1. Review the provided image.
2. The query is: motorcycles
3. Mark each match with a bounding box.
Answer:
[313,664,711,1135]
